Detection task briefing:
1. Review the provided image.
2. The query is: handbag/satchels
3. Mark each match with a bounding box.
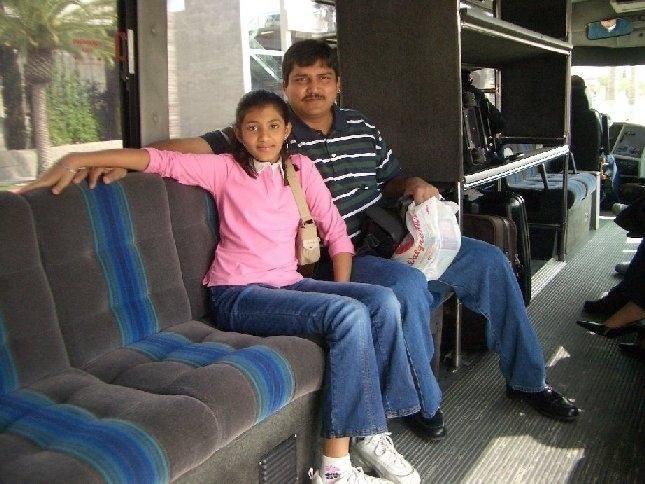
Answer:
[363,206,406,259]
[297,219,321,266]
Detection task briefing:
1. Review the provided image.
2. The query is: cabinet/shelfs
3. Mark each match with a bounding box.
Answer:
[337,0,574,375]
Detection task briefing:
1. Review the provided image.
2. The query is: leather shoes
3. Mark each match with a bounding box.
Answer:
[585,296,613,315]
[406,408,447,440]
[506,387,579,420]
[615,263,629,276]
[576,317,645,358]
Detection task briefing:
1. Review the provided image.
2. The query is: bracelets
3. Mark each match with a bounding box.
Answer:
[55,156,78,176]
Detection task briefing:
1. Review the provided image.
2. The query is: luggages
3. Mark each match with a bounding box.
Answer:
[443,190,532,346]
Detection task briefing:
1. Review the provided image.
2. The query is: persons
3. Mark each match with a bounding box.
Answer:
[576,176,645,356]
[72,39,579,439]
[14,90,424,484]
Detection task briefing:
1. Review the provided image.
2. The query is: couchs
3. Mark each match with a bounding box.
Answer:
[501,142,601,261]
[0,172,457,483]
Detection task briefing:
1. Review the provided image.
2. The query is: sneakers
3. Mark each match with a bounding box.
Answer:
[307,432,421,484]
[612,202,629,217]
